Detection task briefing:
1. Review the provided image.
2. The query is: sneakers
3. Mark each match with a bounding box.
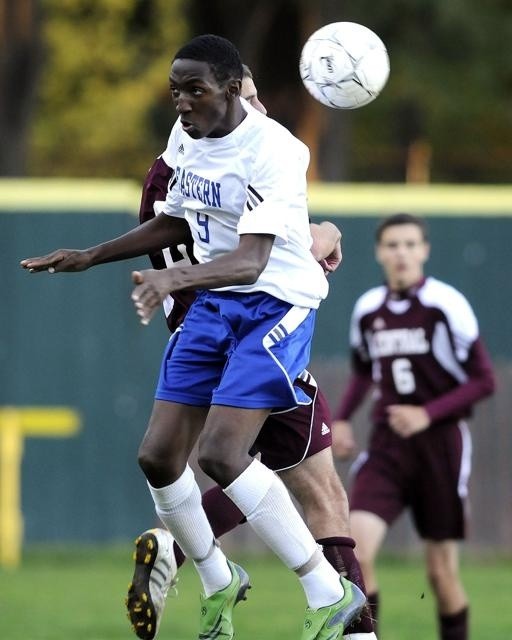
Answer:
[125,528,178,639]
[302,576,367,640]
[199,559,251,640]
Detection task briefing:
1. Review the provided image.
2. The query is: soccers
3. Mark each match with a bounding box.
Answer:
[299,22,390,108]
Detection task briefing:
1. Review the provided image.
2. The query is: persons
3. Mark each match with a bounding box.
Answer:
[330,212,495,640]
[124,64,379,640]
[20,34,369,640]
